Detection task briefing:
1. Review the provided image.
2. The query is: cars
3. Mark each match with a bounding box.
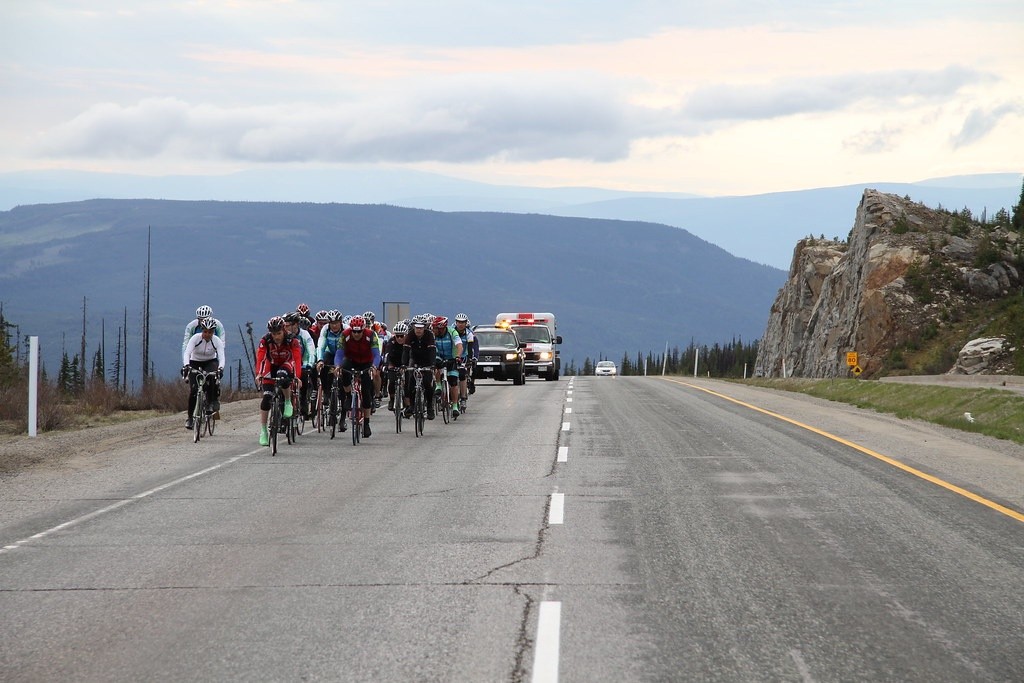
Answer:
[594,360,618,376]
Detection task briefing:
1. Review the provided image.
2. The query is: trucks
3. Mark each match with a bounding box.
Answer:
[495,313,563,381]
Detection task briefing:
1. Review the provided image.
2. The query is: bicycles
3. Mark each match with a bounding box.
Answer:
[258,358,477,456]
[180,366,222,443]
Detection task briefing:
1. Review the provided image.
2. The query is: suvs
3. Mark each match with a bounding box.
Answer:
[470,324,527,385]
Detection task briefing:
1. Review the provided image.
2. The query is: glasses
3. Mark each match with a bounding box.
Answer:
[396,335,404,338]
[352,330,362,333]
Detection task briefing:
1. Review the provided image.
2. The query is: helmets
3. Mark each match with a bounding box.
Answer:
[201,316,217,331]
[267,304,470,332]
[195,305,213,319]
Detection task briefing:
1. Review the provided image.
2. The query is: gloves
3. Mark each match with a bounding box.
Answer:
[217,369,224,379]
[181,368,188,377]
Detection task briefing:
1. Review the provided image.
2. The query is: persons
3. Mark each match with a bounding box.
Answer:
[537,329,548,340]
[254,302,392,445]
[381,313,479,420]
[182,305,225,365]
[180,316,225,430]
[499,335,511,345]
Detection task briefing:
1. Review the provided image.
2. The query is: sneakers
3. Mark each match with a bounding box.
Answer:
[259,431,269,445]
[185,418,193,428]
[207,404,214,412]
[283,402,294,417]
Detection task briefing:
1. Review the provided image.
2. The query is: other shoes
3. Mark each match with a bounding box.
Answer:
[300,402,307,414]
[405,405,413,416]
[388,401,394,410]
[452,404,459,416]
[322,401,329,406]
[339,422,346,432]
[342,397,351,410]
[383,389,388,397]
[435,383,442,394]
[469,383,476,394]
[461,399,467,410]
[427,408,435,420]
[373,396,380,407]
[364,426,371,437]
